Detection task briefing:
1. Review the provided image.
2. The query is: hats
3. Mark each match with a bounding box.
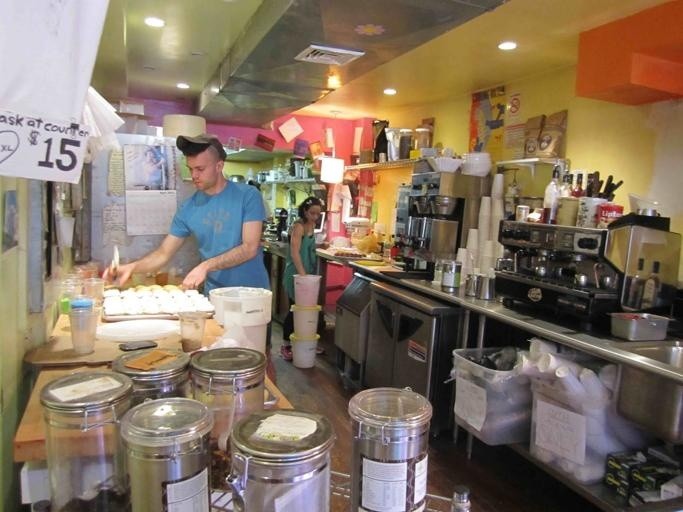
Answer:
[176,133,226,161]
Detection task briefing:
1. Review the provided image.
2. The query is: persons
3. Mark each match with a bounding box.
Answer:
[249,178,273,221]
[280,196,324,361]
[101,133,275,386]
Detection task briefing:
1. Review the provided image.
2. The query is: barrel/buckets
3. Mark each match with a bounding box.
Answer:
[290,305,322,336]
[210,286,273,356]
[289,332,321,369]
[293,274,322,306]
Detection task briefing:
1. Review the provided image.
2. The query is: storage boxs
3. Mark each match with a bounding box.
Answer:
[452,345,675,487]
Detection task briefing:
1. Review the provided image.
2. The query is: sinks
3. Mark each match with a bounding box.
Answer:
[616,341,683,445]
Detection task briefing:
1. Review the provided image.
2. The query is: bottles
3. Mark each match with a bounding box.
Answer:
[382,233,392,263]
[39,370,132,512]
[640,261,661,309]
[345,385,436,512]
[224,409,332,512]
[370,118,432,165]
[188,345,266,489]
[112,350,190,408]
[441,262,461,294]
[541,169,584,225]
[622,257,645,309]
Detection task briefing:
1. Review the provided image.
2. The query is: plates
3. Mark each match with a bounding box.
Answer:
[228,175,245,184]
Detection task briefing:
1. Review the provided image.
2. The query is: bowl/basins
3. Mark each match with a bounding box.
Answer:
[460,152,492,177]
[427,156,463,173]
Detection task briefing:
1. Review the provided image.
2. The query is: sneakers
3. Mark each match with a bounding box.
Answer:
[316,347,325,354]
[278,344,293,360]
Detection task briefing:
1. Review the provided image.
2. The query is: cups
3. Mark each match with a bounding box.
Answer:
[636,208,660,217]
[179,312,206,353]
[465,273,479,297]
[59,263,104,355]
[516,337,647,483]
[476,274,496,300]
[292,160,312,178]
[455,174,506,285]
[574,196,608,229]
